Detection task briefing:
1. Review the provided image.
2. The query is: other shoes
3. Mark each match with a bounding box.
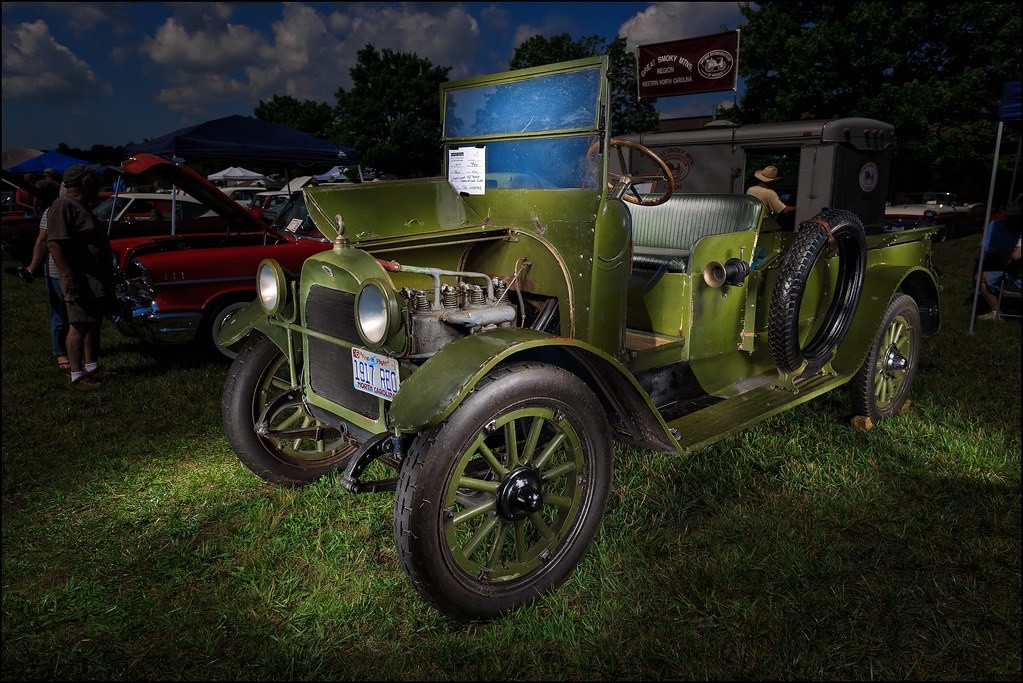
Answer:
[976,311,1000,321]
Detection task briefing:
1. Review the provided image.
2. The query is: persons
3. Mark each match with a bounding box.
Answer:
[975,236,1022,321]
[747,166,796,219]
[17,165,119,390]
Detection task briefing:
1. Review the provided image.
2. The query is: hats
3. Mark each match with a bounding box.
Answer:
[63,163,101,185]
[44,168,58,176]
[754,165,784,183]
[999,200,1023,215]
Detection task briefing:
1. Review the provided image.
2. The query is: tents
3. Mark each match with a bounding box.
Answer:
[107,115,399,241]
[970,81,1023,335]
[1,148,106,176]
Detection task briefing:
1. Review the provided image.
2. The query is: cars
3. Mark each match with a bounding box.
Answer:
[0,153,355,365]
[208,54,943,620]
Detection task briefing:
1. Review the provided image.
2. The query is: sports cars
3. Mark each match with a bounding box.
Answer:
[885,192,989,236]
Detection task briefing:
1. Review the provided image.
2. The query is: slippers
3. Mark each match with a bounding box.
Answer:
[56,359,71,370]
[84,366,117,381]
[67,375,103,391]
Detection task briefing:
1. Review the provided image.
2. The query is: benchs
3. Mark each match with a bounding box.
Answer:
[619,192,763,273]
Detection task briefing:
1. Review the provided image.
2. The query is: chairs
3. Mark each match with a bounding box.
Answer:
[994,260,1022,327]
